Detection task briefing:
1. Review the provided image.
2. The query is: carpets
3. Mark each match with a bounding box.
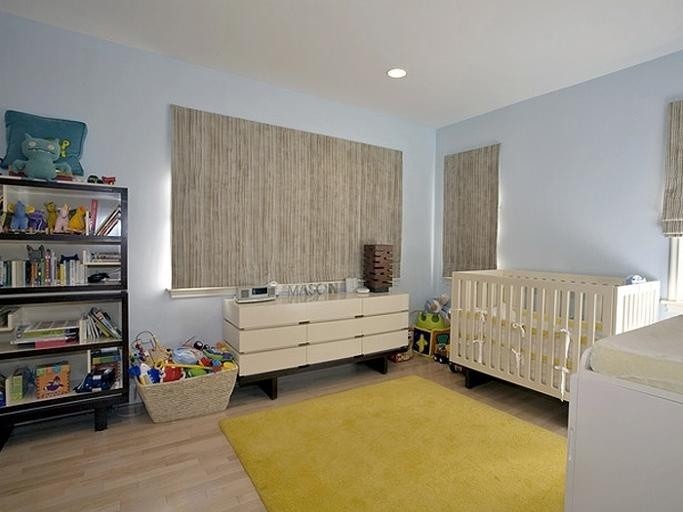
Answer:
[219,374,567,512]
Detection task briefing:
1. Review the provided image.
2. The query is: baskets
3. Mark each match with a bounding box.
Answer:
[133,345,239,424]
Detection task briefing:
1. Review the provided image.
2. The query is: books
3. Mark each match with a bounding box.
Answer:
[11,307,122,344]
[0,247,122,287]
[85,199,121,237]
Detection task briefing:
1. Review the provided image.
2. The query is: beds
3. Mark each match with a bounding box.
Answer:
[446,268,661,404]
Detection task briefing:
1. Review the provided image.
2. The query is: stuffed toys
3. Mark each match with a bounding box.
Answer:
[128,332,236,384]
[0,196,86,235]
[12,132,71,182]
[421,293,451,322]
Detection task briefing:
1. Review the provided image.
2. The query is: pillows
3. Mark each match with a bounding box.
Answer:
[2,110,88,176]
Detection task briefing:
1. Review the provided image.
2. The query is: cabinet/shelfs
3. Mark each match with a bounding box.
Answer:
[0,174,130,453]
[221,290,410,400]
